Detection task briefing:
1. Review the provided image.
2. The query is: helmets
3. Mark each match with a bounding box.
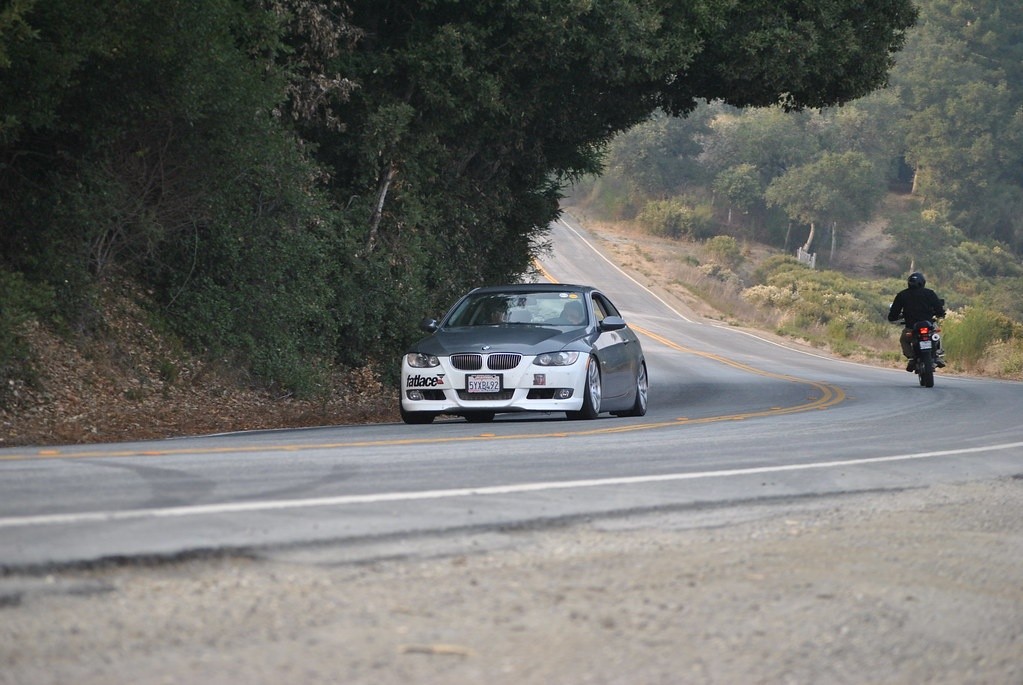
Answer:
[908,272,926,287]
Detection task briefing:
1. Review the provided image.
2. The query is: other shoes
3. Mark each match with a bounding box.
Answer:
[906,360,914,372]
[935,358,946,367]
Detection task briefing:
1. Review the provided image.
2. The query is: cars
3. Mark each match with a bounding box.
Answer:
[398,283,649,423]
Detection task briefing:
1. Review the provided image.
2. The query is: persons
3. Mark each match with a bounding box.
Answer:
[485,301,508,323]
[889,273,946,371]
[562,300,582,324]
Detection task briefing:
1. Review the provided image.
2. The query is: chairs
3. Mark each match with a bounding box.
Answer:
[509,310,531,322]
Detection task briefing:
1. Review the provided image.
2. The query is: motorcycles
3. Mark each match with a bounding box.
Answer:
[889,298,946,388]
